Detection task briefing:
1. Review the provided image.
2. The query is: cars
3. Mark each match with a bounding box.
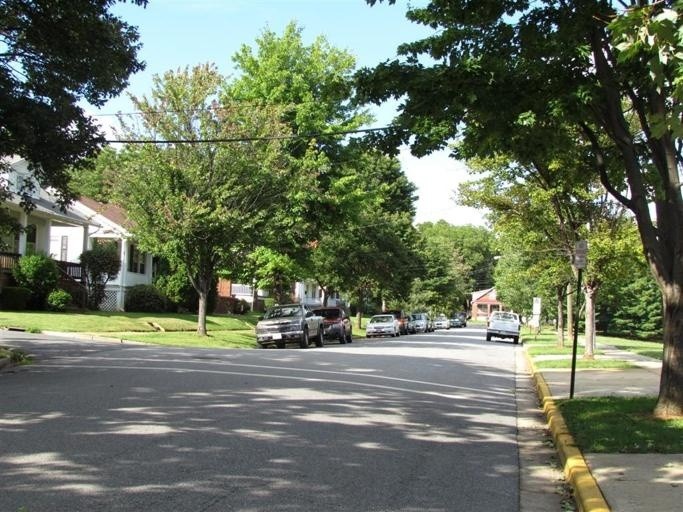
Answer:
[366,310,435,337]
[313,308,352,344]
[433,313,450,329]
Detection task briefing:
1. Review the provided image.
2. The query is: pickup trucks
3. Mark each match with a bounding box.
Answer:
[255,304,324,348]
[486,311,519,343]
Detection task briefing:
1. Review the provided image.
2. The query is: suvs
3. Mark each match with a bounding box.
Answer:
[450,313,466,328]
